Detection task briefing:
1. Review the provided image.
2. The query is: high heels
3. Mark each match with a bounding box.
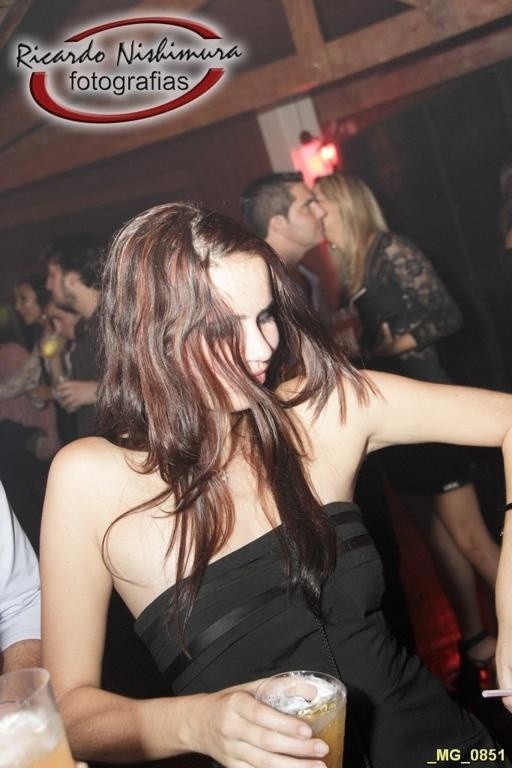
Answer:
[450,628,498,687]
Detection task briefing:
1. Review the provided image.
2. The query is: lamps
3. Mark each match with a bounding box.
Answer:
[290,126,339,192]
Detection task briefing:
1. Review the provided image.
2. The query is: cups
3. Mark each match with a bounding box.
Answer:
[250,670,348,766]
[38,333,62,359]
[0,668,80,768]
[329,305,368,354]
[50,374,82,415]
[23,380,46,412]
